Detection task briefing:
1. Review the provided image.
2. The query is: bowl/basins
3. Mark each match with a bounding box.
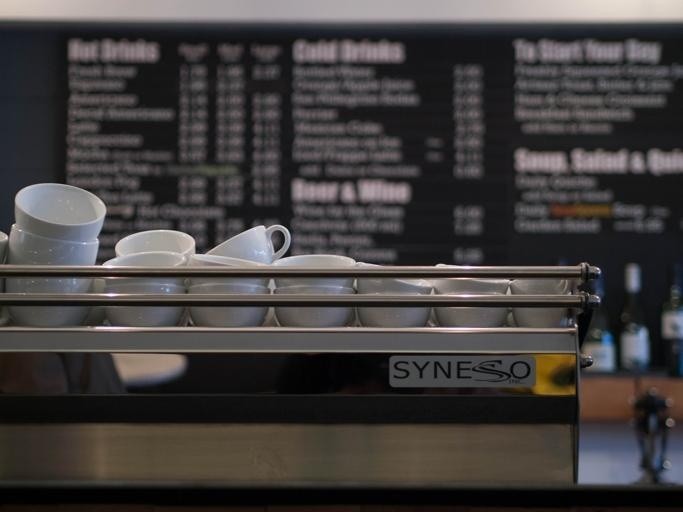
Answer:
[270,253,355,326]
[506,276,571,327]
[101,252,185,324]
[115,229,196,258]
[4,184,107,327]
[206,224,291,326]
[354,263,435,330]
[432,263,510,325]
[185,254,273,325]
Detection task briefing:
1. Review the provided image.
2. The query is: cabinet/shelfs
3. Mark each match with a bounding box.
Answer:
[1,263,602,490]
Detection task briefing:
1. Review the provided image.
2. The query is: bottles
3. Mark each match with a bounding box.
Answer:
[580,264,683,378]
